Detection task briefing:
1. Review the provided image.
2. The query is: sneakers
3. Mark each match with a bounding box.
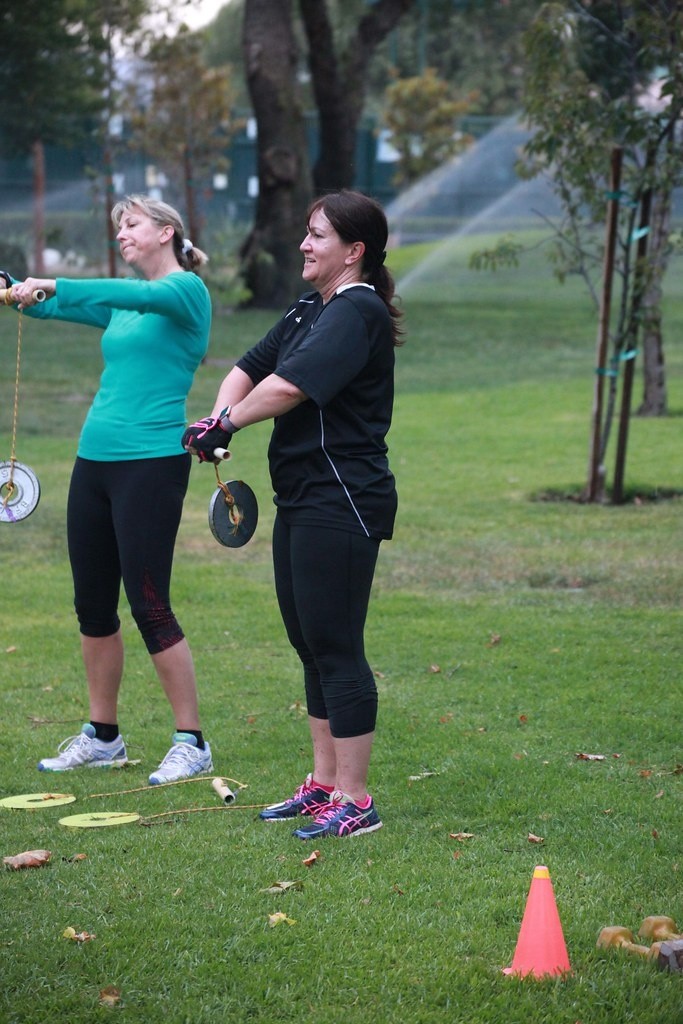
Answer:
[148,733,214,786]
[38,723,129,772]
[293,791,383,841]
[260,773,331,821]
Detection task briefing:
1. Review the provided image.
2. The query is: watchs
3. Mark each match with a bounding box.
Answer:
[218,405,240,434]
[0,270,13,289]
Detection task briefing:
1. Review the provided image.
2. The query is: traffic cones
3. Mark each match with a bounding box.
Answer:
[501,865,575,981]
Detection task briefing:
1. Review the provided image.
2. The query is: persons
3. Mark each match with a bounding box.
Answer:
[0,194,218,785]
[181,188,409,840]
